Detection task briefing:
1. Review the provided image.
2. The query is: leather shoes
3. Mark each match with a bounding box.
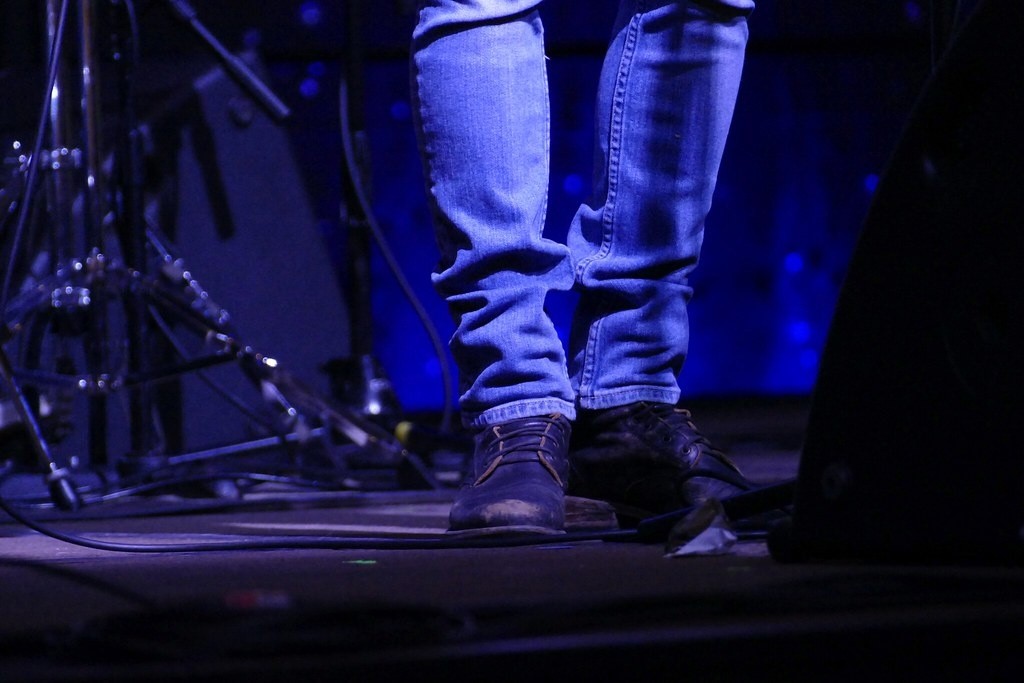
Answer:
[444,412,573,540]
[568,402,756,517]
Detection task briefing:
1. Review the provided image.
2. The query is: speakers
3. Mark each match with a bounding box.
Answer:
[774,0,1024,576]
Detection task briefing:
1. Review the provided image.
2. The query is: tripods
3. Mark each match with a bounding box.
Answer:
[0,0,440,514]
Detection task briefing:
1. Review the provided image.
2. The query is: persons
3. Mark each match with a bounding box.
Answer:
[410,0,755,540]
